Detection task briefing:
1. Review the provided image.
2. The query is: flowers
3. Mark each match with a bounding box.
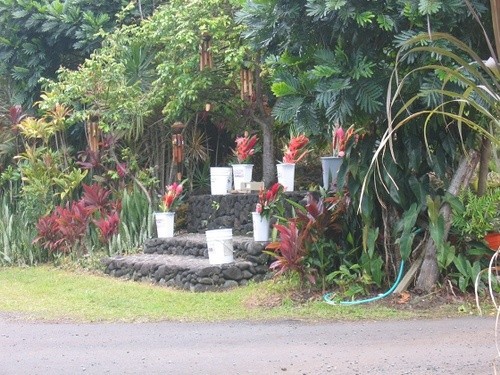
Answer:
[276,126,315,165]
[331,117,366,158]
[256,182,286,222]
[158,178,189,213]
[230,131,265,163]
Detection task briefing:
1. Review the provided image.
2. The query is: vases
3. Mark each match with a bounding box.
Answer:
[154,211,175,238]
[252,211,270,242]
[276,163,296,192]
[232,164,254,194]
[321,157,348,193]
